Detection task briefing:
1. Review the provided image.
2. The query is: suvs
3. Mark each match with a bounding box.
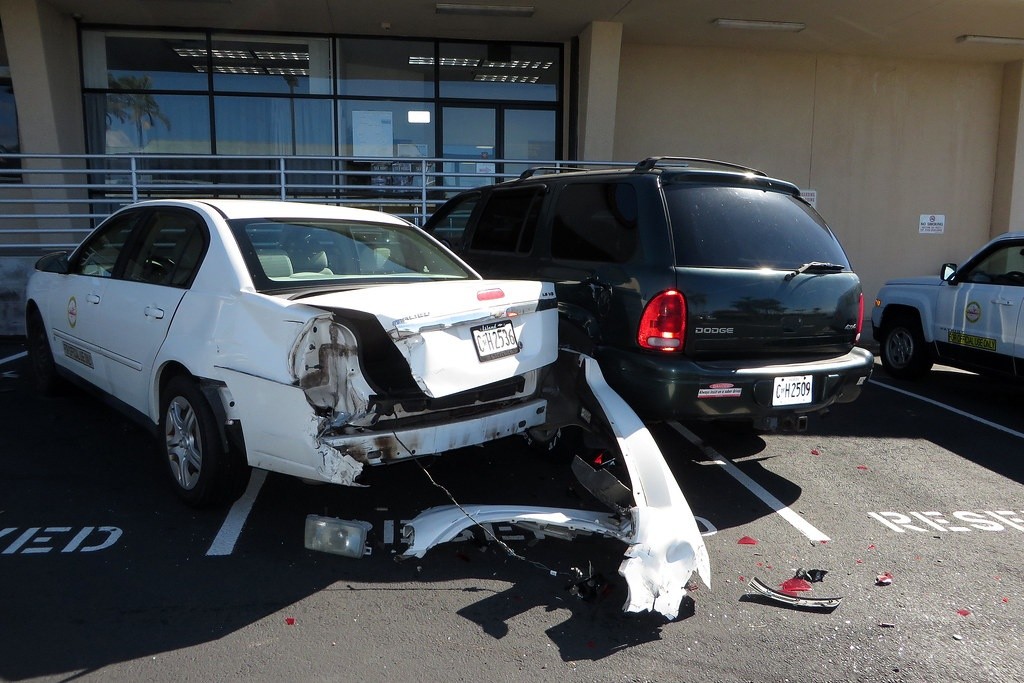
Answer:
[870,230,1024,382]
[419,154,876,435]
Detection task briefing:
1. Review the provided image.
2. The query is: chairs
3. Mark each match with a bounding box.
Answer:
[360,244,407,274]
[286,237,334,275]
[170,225,204,284]
[257,248,294,277]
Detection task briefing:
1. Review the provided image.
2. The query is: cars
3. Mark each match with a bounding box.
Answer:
[24,197,561,510]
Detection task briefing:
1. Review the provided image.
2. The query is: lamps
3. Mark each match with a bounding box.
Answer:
[711,18,805,33]
[955,35,1024,46]
[435,3,535,17]
